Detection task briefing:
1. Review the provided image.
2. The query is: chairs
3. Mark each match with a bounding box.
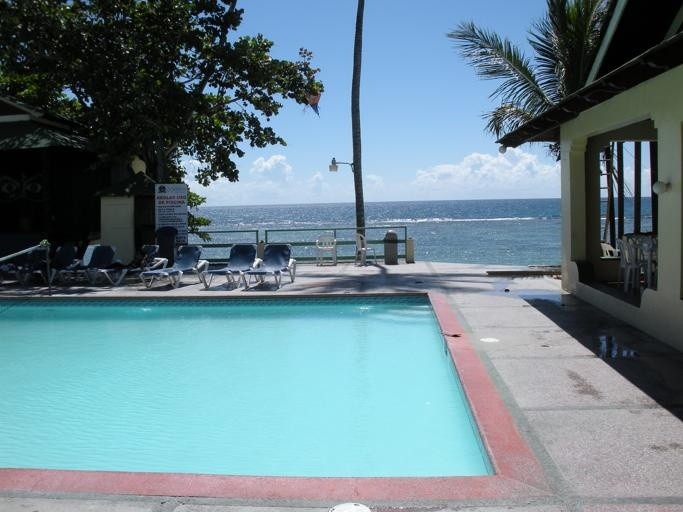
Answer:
[600,237,657,294]
[353,234,378,267]
[32,244,297,290]
[316,236,338,266]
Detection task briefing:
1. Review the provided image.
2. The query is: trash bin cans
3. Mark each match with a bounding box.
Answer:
[383,230,398,265]
